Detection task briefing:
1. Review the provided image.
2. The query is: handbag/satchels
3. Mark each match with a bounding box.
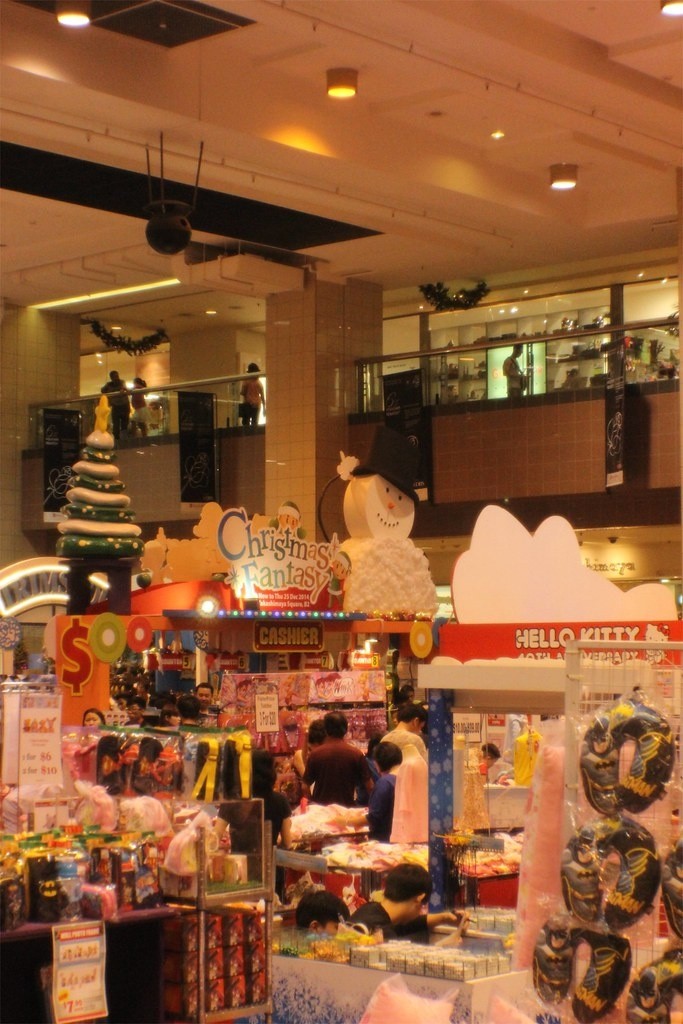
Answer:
[238,402,252,417]
[520,375,527,389]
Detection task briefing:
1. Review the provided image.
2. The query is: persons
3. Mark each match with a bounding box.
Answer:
[481,743,514,786]
[503,343,527,398]
[295,891,349,939]
[82,708,106,727]
[121,377,148,437]
[240,363,265,425]
[301,685,430,838]
[101,370,130,441]
[350,863,469,950]
[212,748,292,906]
[107,657,225,731]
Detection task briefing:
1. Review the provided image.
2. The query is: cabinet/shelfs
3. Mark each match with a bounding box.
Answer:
[625,322,679,384]
[0,671,683,1024]
[430,304,612,404]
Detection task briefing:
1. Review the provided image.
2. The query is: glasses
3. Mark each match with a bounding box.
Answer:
[125,707,142,713]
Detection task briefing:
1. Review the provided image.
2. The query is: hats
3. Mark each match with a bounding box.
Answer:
[514,345,523,353]
[247,363,260,372]
[351,427,421,507]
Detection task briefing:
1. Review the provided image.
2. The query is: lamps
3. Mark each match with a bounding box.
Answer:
[660,0,683,14]
[549,163,577,190]
[55,0,91,25]
[327,68,358,97]
